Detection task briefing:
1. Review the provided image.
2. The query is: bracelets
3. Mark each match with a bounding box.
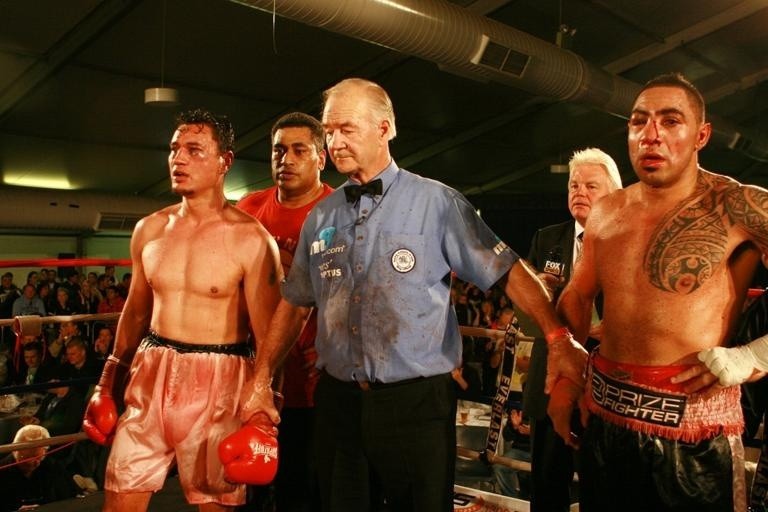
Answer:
[546,327,570,345]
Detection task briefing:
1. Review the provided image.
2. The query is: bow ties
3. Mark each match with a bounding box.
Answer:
[342,178,383,203]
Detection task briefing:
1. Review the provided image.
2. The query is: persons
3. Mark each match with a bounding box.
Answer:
[62,271,81,294]
[78,281,103,315]
[235,112,337,512]
[94,328,114,360]
[56,336,106,384]
[238,78,589,512]
[0,272,23,319]
[38,270,61,289]
[515,148,623,512]
[48,287,82,315]
[0,425,97,512]
[48,319,89,358]
[27,271,39,288]
[83,265,132,297]
[452,273,531,498]
[547,74,768,512]
[12,284,46,317]
[98,285,126,313]
[83,109,285,512]
[19,379,84,437]
[16,341,56,385]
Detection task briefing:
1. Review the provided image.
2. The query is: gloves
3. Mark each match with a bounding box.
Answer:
[698,335,768,388]
